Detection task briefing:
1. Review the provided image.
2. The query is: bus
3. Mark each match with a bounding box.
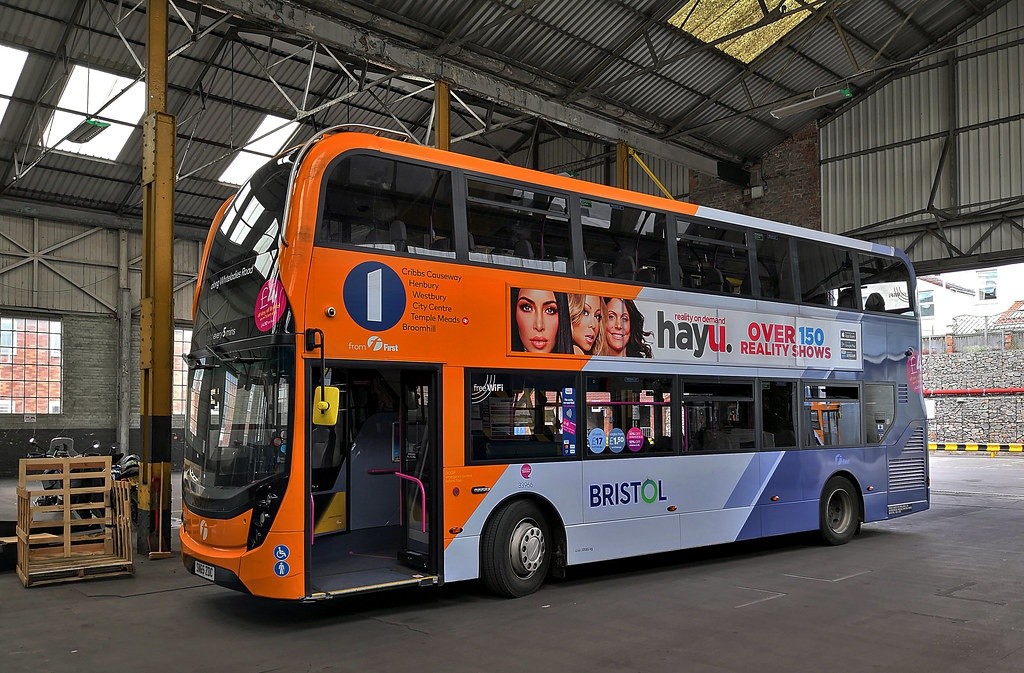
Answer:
[176,123,939,606]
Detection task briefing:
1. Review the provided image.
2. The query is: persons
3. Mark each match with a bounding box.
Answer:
[511,287,575,355]
[569,294,609,355]
[602,297,655,359]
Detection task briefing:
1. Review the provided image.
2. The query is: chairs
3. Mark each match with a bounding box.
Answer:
[380,217,889,314]
[469,418,800,462]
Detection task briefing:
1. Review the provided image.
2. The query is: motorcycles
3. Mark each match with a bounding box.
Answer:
[26,434,140,529]
